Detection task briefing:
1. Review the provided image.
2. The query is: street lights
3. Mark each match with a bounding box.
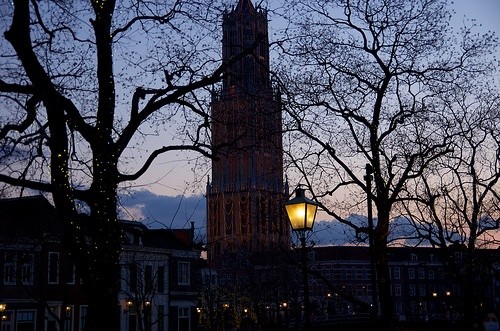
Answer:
[283,184,322,331]
